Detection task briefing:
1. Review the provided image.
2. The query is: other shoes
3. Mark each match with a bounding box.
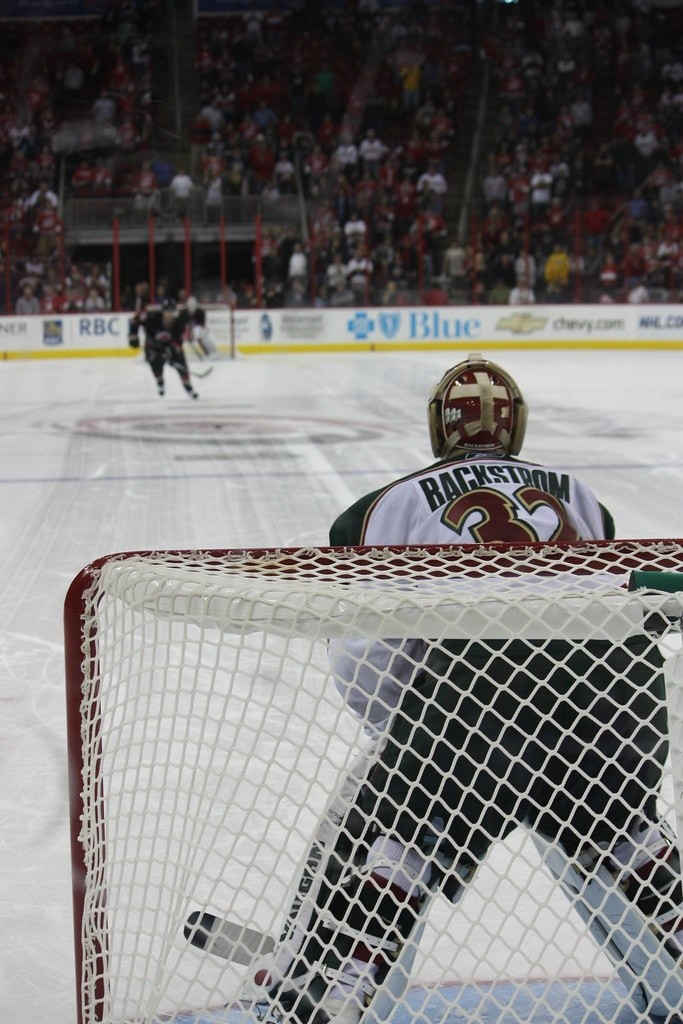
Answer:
[184,384,198,398]
[158,381,165,396]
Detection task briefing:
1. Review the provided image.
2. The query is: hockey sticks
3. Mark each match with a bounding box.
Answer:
[175,738,394,976]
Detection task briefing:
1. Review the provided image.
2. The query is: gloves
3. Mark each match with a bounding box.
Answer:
[130,334,139,348]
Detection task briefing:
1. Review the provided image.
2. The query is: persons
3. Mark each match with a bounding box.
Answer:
[247,358,683,1024]
[0,0,683,400]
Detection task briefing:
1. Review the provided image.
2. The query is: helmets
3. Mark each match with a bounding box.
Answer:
[427,353,528,460]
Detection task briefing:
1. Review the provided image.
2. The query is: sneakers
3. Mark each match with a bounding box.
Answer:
[272,998,360,1024]
